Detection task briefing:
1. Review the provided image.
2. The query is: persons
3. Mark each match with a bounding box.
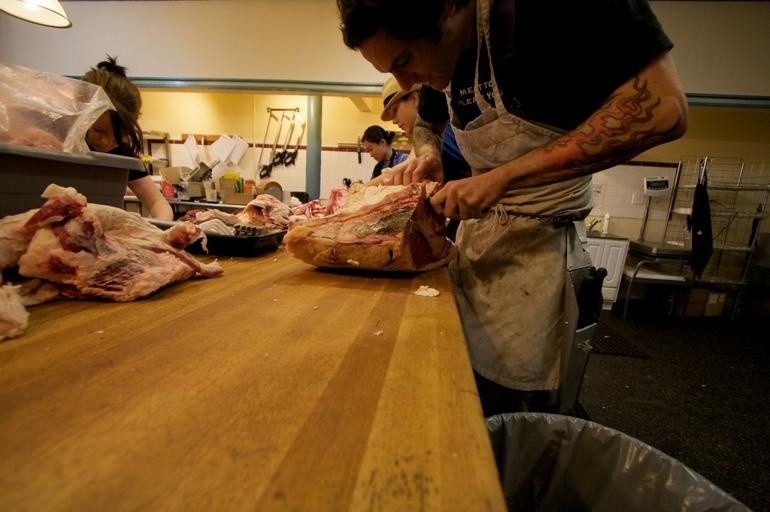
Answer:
[335,0,689,417]
[370,73,422,175]
[43,50,175,223]
[360,126,409,179]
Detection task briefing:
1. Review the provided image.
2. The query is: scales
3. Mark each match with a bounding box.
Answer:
[628,176,693,261]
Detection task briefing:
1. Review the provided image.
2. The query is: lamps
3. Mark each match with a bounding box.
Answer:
[1,0,72,29]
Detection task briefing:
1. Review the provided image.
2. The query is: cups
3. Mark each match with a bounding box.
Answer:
[206,189,217,204]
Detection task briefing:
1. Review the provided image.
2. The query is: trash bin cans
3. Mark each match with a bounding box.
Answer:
[485,412,753,512]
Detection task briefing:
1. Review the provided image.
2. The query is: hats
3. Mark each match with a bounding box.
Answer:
[380,75,425,122]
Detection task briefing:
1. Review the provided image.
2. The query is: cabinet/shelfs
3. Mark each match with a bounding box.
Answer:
[667,152,769,325]
